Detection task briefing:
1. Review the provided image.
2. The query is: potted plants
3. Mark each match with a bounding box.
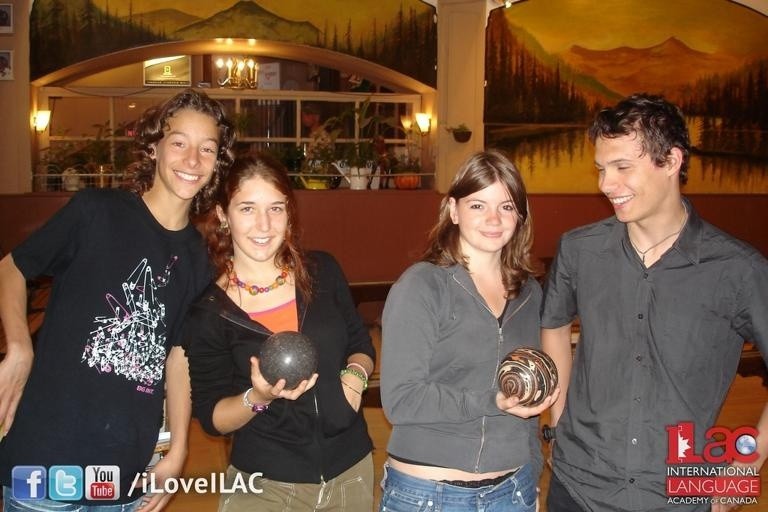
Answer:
[339,94,405,193]
[392,154,425,187]
[90,134,117,188]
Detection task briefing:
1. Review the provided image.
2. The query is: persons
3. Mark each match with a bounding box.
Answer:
[378,150,561,512]
[1,87,238,512]
[540,92,767,512]
[180,150,377,511]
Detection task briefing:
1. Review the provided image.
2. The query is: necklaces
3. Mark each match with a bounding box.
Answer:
[227,254,290,296]
[630,217,686,263]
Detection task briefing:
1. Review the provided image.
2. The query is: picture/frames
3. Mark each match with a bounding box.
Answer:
[141,54,194,88]
[0,48,15,80]
[0,2,14,35]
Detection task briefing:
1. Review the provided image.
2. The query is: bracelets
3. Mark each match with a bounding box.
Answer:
[338,368,368,391]
[347,363,369,381]
[341,380,361,395]
[243,387,272,414]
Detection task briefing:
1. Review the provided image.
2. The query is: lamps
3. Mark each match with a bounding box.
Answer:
[413,110,434,139]
[208,55,265,91]
[34,108,53,134]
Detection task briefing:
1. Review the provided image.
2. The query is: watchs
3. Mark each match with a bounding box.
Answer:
[542,424,557,444]
